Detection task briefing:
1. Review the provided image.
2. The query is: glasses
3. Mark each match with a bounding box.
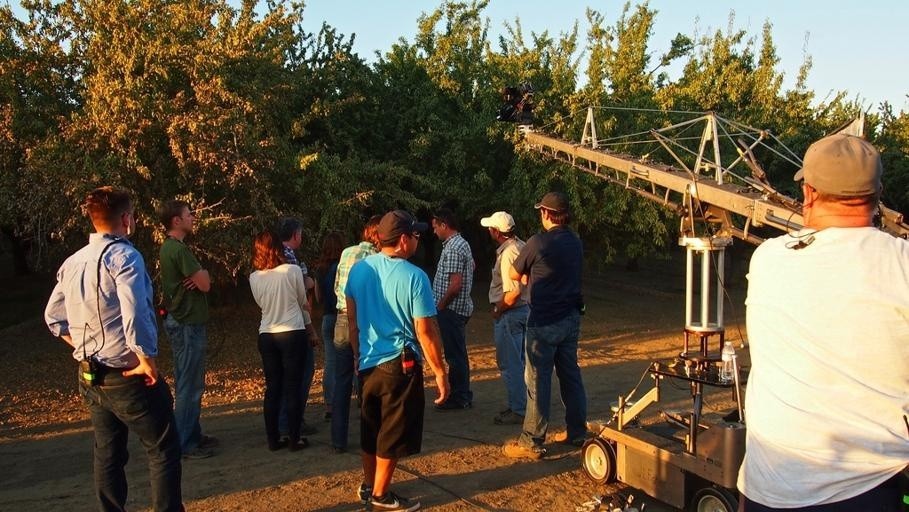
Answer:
[409,232,420,239]
[431,213,446,225]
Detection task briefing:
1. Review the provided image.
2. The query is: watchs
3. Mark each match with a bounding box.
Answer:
[493,305,502,314]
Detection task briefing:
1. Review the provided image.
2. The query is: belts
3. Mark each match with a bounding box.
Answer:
[338,308,348,314]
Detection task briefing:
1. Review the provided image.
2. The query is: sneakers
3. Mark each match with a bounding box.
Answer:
[495,411,525,424]
[333,443,345,453]
[358,485,374,505]
[300,422,319,435]
[196,436,218,447]
[367,492,421,511]
[180,443,212,459]
[554,430,585,445]
[434,396,471,411]
[502,439,542,458]
[324,411,332,420]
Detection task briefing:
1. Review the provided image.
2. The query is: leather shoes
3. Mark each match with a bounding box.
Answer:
[270,437,289,451]
[290,437,308,451]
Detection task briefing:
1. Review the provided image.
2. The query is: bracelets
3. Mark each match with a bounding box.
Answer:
[352,353,360,361]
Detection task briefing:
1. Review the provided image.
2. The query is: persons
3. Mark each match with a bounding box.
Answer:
[343,208,453,512]
[419,213,475,412]
[44,185,186,512]
[330,214,383,455]
[500,191,588,463]
[275,217,319,438]
[735,133,909,512]
[247,228,319,453]
[488,211,530,427]
[312,230,363,421]
[159,200,222,461]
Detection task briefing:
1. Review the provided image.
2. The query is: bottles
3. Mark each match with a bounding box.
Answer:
[720,340,737,380]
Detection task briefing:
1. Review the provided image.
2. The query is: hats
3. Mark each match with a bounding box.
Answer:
[377,210,415,243]
[535,192,568,212]
[481,211,515,232]
[793,135,884,198]
